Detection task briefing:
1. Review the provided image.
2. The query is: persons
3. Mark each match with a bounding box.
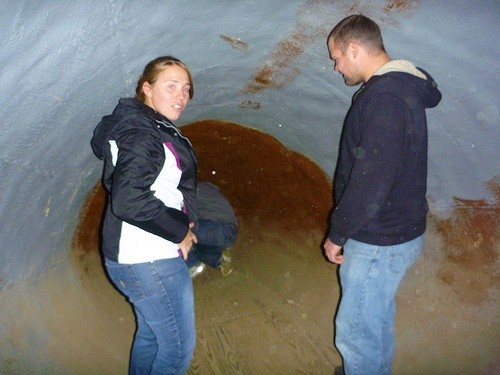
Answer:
[183,180,240,279]
[90,56,200,375]
[322,14,442,375]
